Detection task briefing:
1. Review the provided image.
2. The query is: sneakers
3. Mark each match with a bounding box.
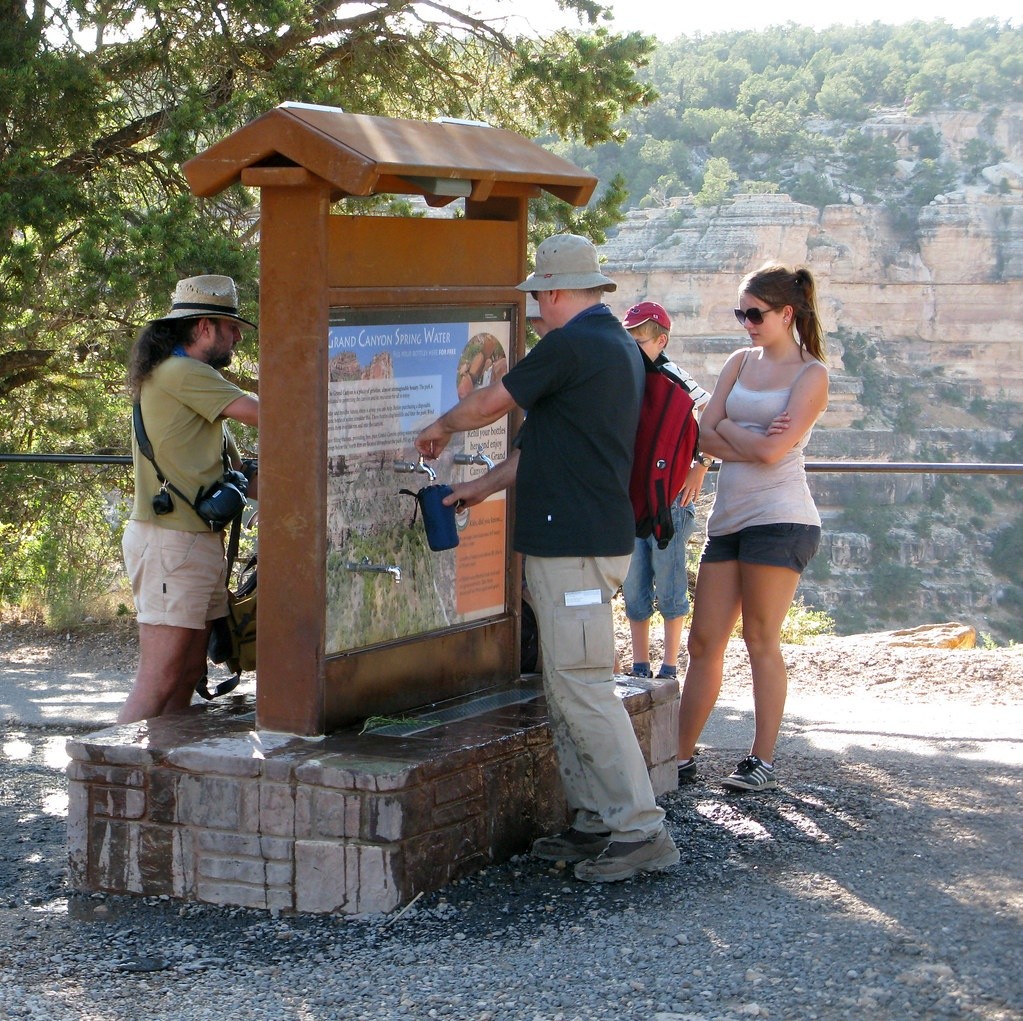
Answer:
[678,757,697,784]
[575,825,680,881]
[532,825,613,861]
[722,754,777,791]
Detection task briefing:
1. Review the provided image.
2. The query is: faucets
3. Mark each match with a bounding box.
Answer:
[347,555,402,585]
[392,451,440,481]
[452,442,496,473]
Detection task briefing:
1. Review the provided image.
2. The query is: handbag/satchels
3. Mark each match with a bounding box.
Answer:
[224,573,256,673]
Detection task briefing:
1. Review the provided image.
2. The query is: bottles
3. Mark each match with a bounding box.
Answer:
[417,482,459,552]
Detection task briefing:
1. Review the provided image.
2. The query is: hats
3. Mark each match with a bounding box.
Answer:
[515,233,616,294]
[521,273,542,317]
[148,275,258,330]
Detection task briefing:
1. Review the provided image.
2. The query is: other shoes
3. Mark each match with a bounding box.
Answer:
[658,672,678,679]
[630,670,653,677]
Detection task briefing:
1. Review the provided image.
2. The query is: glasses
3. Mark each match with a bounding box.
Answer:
[734,305,782,326]
[637,336,654,347]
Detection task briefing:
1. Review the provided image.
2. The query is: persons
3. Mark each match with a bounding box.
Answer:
[415,234,681,881]
[625,301,713,678]
[118,275,259,729]
[677,264,830,791]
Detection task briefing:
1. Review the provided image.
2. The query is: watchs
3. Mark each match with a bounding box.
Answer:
[697,456,715,467]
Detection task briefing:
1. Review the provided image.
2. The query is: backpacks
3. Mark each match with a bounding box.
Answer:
[635,340,699,550]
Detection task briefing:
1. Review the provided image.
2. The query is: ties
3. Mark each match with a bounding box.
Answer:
[620,302,670,332]
